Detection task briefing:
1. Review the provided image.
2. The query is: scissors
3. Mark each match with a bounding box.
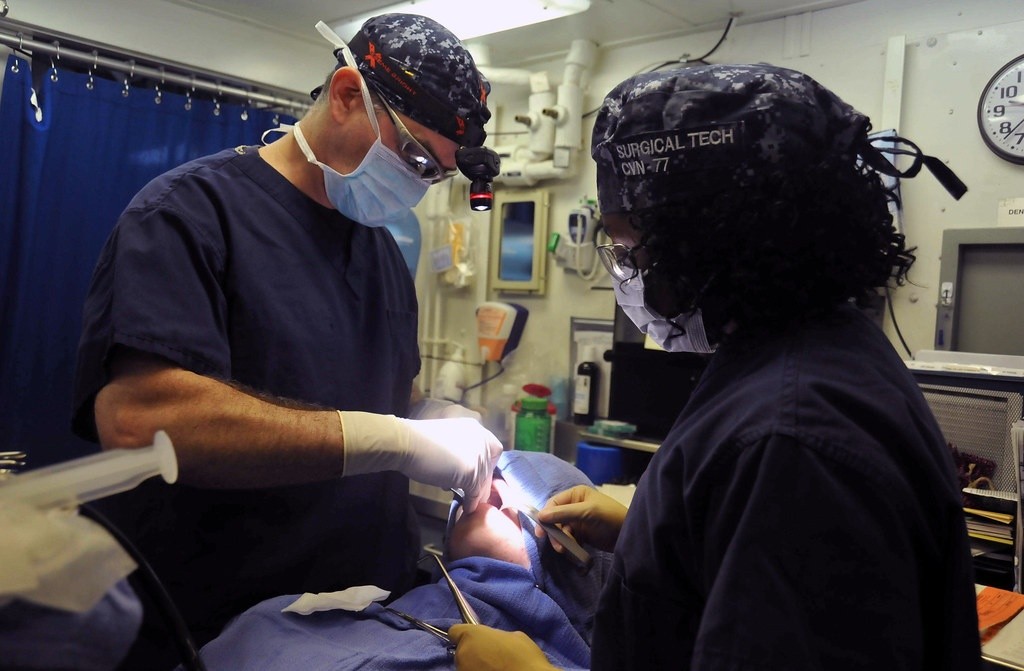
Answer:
[383,608,459,659]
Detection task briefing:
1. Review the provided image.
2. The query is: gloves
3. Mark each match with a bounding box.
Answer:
[408,397,482,424]
[336,407,504,513]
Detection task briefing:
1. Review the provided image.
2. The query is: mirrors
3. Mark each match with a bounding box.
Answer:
[490,188,552,296]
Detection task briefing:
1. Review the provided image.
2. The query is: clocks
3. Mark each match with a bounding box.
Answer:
[977,54,1024,167]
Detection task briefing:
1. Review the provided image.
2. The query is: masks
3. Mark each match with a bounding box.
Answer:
[607,262,736,354]
[291,20,431,229]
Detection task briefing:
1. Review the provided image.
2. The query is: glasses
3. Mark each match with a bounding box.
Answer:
[591,220,655,285]
[359,72,458,186]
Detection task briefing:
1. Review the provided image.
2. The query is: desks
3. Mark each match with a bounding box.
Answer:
[577,430,661,485]
[974,583,1024,671]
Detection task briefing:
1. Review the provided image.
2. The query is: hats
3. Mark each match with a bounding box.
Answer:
[590,66,869,215]
[336,13,491,146]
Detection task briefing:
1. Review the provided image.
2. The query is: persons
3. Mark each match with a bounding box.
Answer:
[451,59,986,671]
[0,9,618,671]
